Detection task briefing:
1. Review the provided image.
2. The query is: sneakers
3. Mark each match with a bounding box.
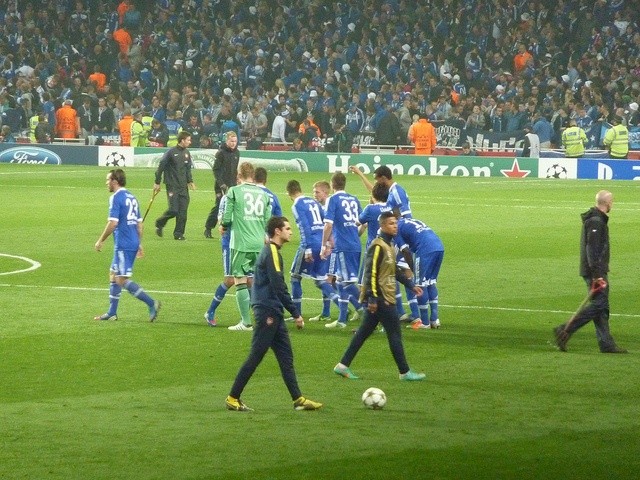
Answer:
[325,320,346,328]
[411,318,422,328]
[309,314,331,321]
[352,326,379,334]
[149,300,161,322]
[600,345,627,353]
[228,322,253,331]
[174,232,185,240]
[399,371,426,380]
[333,363,359,379]
[430,319,440,327]
[204,230,214,238]
[155,225,162,237]
[553,325,568,351]
[350,312,360,321]
[293,396,322,410]
[406,318,431,329]
[204,311,217,327]
[94,313,117,320]
[225,396,254,411]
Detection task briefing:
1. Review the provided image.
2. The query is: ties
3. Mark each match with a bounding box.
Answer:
[152,109,156,116]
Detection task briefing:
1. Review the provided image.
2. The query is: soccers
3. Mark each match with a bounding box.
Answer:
[105,152,126,167]
[546,165,566,178]
[362,387,386,410]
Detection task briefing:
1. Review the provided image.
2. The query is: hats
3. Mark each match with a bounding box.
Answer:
[256,49,264,57]
[21,83,31,91]
[442,72,452,79]
[224,88,232,95]
[255,65,264,74]
[561,75,570,83]
[226,57,234,67]
[629,102,638,110]
[303,51,311,58]
[133,110,142,116]
[348,23,355,31]
[517,36,530,45]
[584,80,592,88]
[368,92,376,99]
[310,90,318,98]
[496,85,505,92]
[249,6,256,14]
[608,115,623,123]
[36,105,44,113]
[521,13,530,21]
[274,53,280,59]
[402,44,411,51]
[186,60,193,68]
[342,64,351,72]
[142,106,153,114]
[174,60,183,65]
[454,74,460,82]
[391,56,397,62]
[324,22,332,28]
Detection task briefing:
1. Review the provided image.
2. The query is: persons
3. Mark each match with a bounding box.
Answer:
[284,178,333,323]
[553,189,627,352]
[394,209,444,328]
[228,162,270,331]
[153,131,197,239]
[334,212,427,382]
[307,181,361,320]
[254,168,282,243]
[222,216,323,412]
[204,130,240,238]
[94,169,162,322]
[356,182,399,331]
[349,164,420,323]
[204,188,234,327]
[320,170,367,329]
